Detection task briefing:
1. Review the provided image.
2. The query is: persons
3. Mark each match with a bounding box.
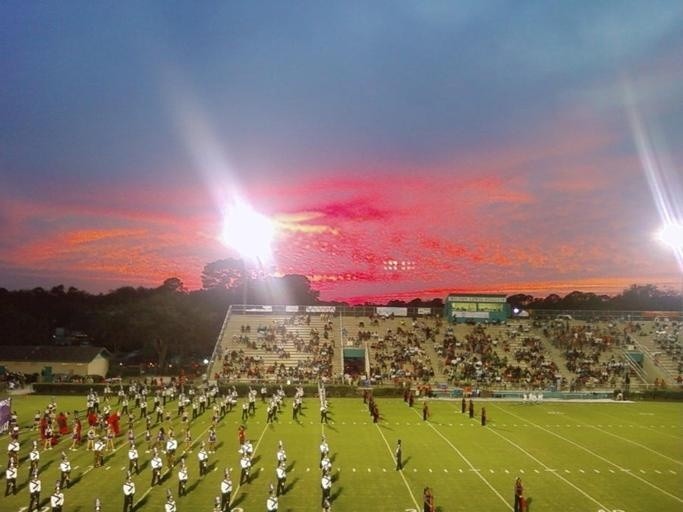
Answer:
[394,438,402,471]
[514,477,527,512]
[6,386,332,511]
[422,487,434,512]
[131,359,202,385]
[423,402,427,421]
[461,398,486,425]
[363,390,378,422]
[404,390,413,407]
[53,374,95,383]
[213,311,682,401]
